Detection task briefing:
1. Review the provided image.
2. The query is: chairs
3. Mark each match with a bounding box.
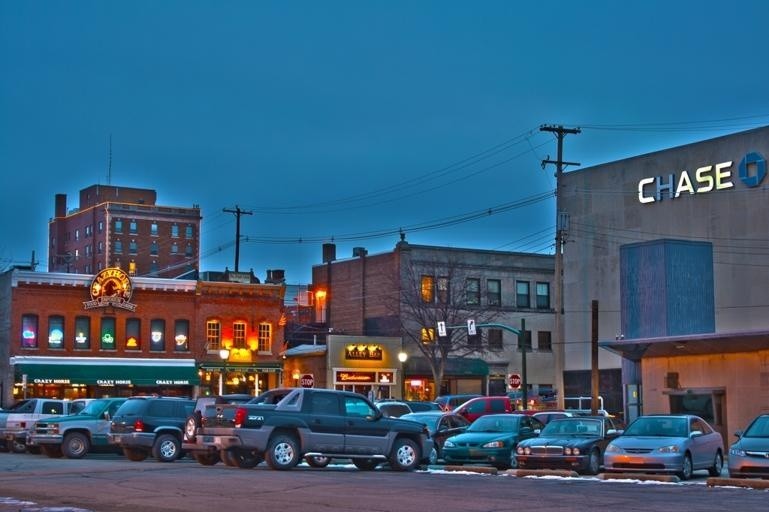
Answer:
[556,424,602,435]
[637,421,687,436]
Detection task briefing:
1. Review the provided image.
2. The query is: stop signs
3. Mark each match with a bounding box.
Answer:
[299,375,315,388]
[507,374,521,391]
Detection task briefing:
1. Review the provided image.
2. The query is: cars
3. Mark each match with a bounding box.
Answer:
[442,414,545,468]
[728,414,769,477]
[513,410,609,426]
[603,415,724,480]
[518,418,627,475]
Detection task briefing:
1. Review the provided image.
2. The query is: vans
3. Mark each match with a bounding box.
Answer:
[434,395,482,411]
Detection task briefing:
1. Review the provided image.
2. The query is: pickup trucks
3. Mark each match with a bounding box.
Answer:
[444,397,562,423]
[0,399,72,453]
[202,387,431,470]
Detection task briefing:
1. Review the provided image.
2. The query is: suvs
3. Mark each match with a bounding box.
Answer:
[108,396,196,462]
[32,398,127,458]
